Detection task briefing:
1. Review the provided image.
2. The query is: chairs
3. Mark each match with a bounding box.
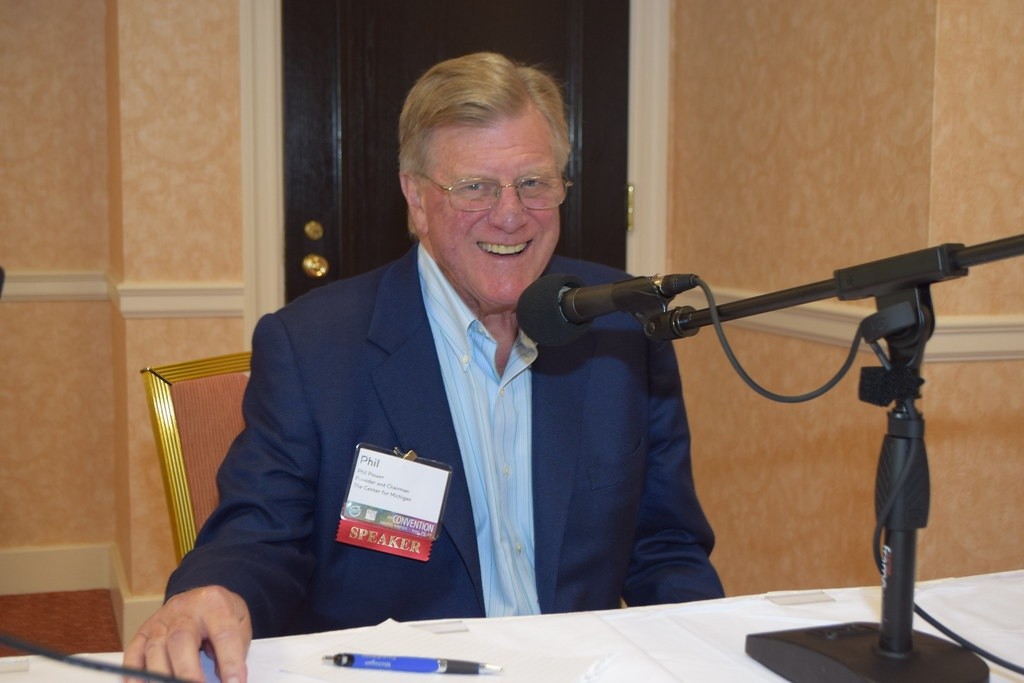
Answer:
[139,351,252,561]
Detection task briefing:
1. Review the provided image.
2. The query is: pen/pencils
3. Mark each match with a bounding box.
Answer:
[322,653,503,677]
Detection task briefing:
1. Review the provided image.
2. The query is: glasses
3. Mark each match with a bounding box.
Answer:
[413,170,574,212]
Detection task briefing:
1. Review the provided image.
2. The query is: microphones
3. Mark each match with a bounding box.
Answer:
[515,274,698,346]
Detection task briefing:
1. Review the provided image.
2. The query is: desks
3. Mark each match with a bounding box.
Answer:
[0,568,1024,683]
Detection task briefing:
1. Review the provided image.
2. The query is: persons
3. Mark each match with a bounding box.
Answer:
[121,51,729,683]
